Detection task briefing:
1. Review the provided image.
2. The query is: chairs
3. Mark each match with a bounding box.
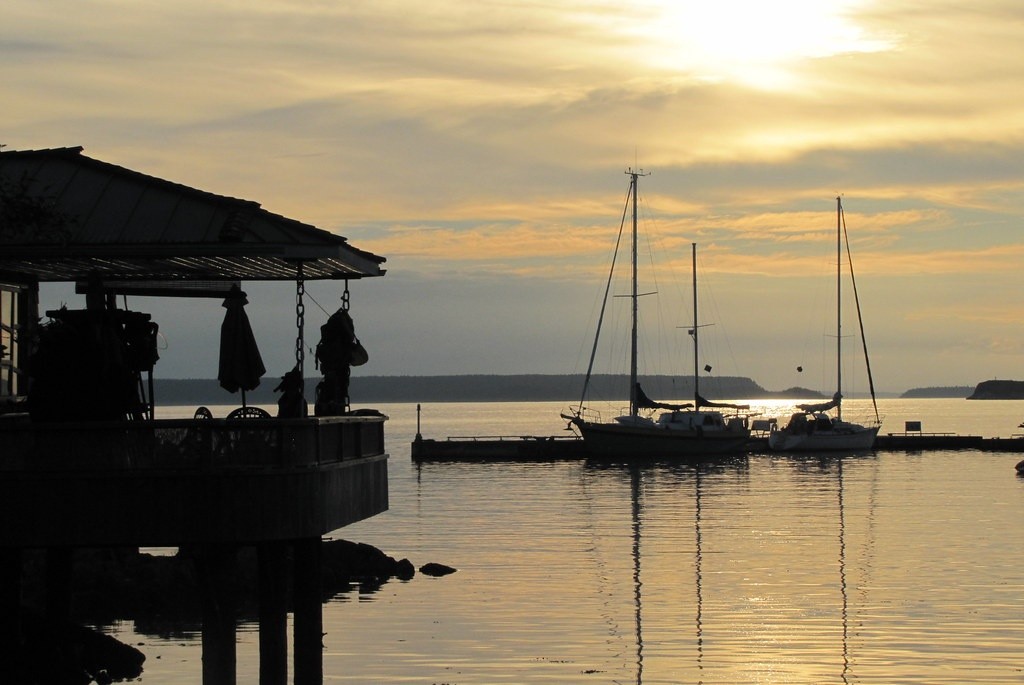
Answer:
[176,406,222,458]
[223,407,274,456]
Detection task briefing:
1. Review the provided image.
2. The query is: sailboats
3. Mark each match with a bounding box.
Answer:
[770,198,881,454]
[560,168,752,461]
[613,243,751,434]
